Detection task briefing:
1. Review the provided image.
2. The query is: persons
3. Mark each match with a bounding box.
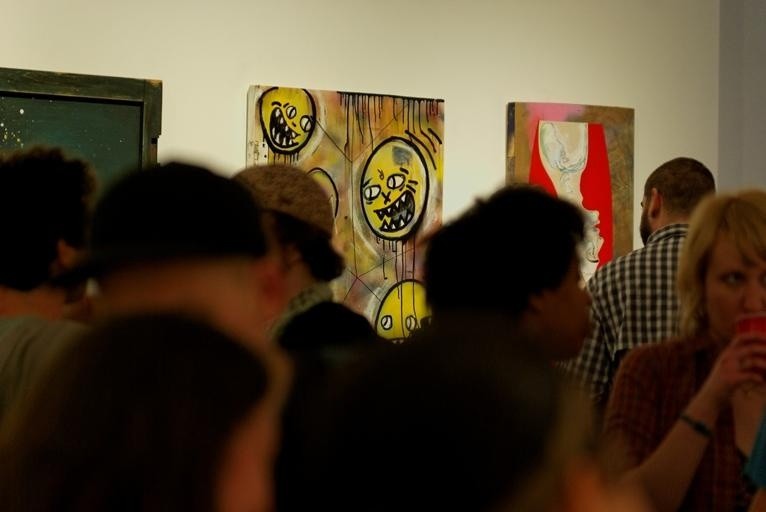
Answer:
[600,186,765,512]
[0,141,650,512]
[553,154,717,478]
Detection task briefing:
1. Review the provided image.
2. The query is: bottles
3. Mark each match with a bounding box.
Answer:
[233,163,335,236]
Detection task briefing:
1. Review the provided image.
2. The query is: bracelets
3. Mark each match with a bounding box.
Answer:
[679,413,715,446]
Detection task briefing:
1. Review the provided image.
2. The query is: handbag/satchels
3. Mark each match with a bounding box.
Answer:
[679,413,715,446]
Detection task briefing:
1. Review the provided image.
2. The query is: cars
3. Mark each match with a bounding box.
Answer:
[733,314,766,385]
